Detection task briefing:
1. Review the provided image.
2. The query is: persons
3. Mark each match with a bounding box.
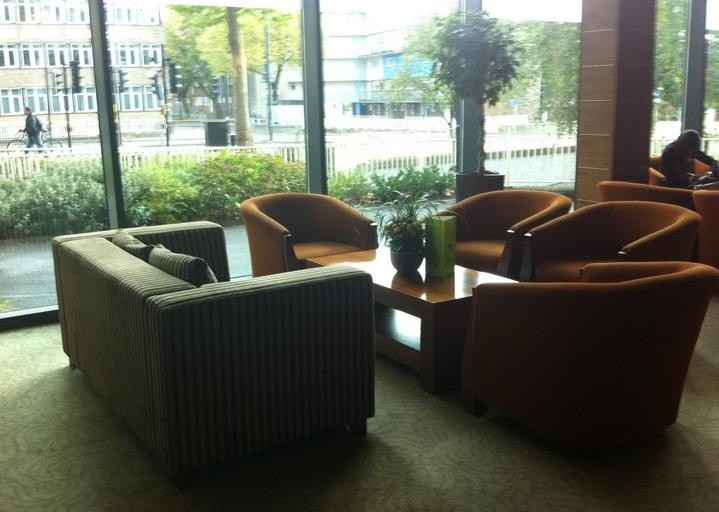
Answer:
[19,106,43,155]
[661,130,719,186]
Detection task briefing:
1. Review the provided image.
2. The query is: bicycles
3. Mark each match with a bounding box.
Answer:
[7,128,64,157]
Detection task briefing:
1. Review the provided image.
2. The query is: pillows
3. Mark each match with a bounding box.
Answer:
[113,227,218,286]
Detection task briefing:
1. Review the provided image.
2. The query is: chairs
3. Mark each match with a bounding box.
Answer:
[462,258,718,449]
[239,193,376,278]
[434,188,572,277]
[596,180,695,213]
[520,198,705,264]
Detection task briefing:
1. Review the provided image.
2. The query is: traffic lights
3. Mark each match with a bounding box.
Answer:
[118,69,129,93]
[56,73,64,93]
[211,76,226,100]
[69,60,84,94]
[149,62,183,100]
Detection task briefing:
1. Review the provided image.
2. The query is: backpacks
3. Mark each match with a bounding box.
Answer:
[37,119,48,132]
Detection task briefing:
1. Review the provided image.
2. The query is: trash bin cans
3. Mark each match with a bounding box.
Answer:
[204,121,230,146]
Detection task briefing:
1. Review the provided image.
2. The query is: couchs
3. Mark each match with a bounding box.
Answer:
[51,219,377,488]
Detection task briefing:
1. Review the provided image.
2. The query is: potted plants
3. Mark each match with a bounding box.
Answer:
[368,189,444,275]
[426,6,527,203]
